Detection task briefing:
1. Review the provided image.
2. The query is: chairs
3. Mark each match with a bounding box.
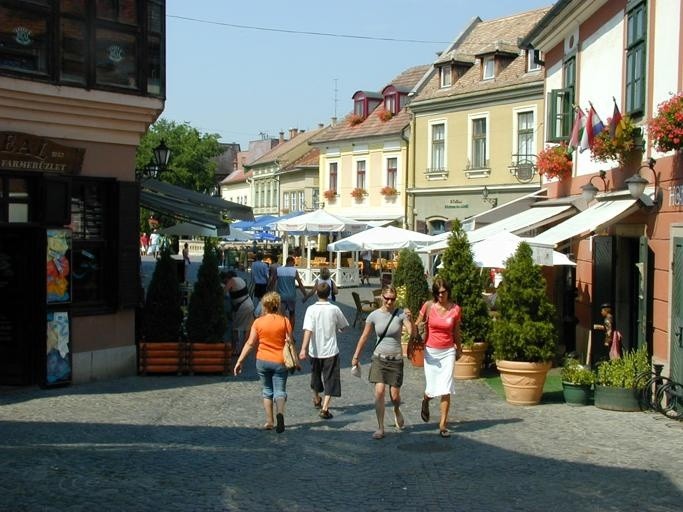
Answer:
[295,256,397,278]
[373,288,382,307]
[351,292,379,327]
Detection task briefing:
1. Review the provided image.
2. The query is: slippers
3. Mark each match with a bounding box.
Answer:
[440,427,450,438]
[421,399,430,423]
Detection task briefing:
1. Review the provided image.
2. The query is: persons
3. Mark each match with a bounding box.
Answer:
[140,229,167,259]
[360,251,374,286]
[234,292,302,433]
[299,282,349,419]
[220,253,338,355]
[414,278,463,437]
[600,303,614,362]
[182,242,190,264]
[351,285,418,438]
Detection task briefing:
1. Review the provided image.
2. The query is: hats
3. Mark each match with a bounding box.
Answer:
[599,302,613,309]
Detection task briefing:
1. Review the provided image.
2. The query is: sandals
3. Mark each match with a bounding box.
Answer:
[372,431,385,440]
[393,408,406,430]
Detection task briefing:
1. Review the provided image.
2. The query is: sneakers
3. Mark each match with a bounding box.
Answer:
[276,412,285,435]
[319,409,333,420]
[263,421,273,431]
[313,396,323,409]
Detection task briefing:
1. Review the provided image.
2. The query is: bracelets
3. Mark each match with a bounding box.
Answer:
[353,356,358,360]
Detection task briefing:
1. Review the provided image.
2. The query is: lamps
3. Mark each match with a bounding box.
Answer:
[134,137,170,179]
[625,158,663,210]
[580,170,606,203]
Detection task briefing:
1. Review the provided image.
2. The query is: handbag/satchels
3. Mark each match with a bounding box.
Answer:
[331,280,336,302]
[409,299,434,344]
[282,315,296,375]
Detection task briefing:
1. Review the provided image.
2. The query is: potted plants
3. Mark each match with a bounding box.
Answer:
[392,247,430,367]
[433,216,486,379]
[593,343,652,412]
[139,237,231,375]
[560,354,596,406]
[492,241,557,404]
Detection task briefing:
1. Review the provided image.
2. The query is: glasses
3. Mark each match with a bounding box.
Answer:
[382,294,396,302]
[435,289,447,296]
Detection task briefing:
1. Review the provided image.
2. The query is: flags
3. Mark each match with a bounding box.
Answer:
[567,105,587,154]
[579,104,604,153]
[608,102,622,142]
